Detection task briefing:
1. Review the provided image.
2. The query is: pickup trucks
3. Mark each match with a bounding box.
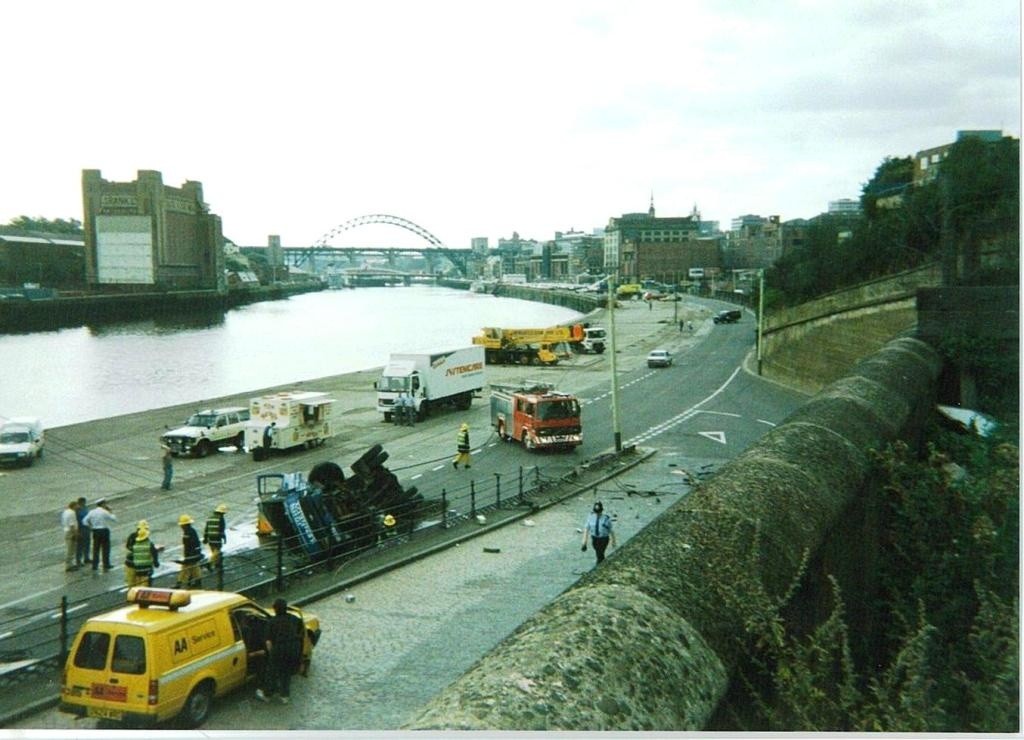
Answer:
[0,417,45,468]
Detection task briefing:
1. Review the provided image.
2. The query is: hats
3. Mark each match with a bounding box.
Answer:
[95,497,105,505]
[594,502,603,513]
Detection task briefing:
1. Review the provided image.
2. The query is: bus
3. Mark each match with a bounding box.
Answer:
[503,274,526,282]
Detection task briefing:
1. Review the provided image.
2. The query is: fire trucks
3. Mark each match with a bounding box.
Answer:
[488,379,584,452]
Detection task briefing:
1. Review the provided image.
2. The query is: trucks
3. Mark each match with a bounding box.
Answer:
[373,345,485,422]
[616,284,639,293]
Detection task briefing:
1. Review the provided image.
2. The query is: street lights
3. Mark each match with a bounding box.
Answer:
[756,268,764,375]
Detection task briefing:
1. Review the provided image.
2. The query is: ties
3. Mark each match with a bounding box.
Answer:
[596,515,599,536]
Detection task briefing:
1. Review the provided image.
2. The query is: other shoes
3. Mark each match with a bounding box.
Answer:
[280,696,289,704]
[65,559,114,571]
[255,689,271,704]
[464,464,470,468]
[453,460,458,469]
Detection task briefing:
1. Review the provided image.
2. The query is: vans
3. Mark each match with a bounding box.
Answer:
[59,589,322,730]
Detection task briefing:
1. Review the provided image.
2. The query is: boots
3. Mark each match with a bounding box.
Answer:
[170,582,182,588]
[191,580,201,587]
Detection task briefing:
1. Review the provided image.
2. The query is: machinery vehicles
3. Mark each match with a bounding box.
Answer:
[568,323,607,354]
[471,324,583,366]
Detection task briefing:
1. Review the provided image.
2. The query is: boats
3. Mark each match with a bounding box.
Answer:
[470,282,483,292]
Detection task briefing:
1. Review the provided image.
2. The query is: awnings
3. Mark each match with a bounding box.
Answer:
[300,398,338,406]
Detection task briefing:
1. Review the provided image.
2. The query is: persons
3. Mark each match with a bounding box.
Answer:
[170,514,202,590]
[256,598,303,703]
[82,497,116,570]
[124,519,160,596]
[452,423,471,470]
[404,393,416,428]
[393,392,405,426]
[263,423,276,460]
[160,445,173,490]
[202,504,227,576]
[649,300,652,311]
[687,319,693,331]
[76,498,94,567]
[61,502,78,572]
[581,500,616,567]
[679,318,684,332]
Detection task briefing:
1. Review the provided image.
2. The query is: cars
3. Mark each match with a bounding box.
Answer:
[647,350,672,368]
[712,310,741,324]
[257,444,426,557]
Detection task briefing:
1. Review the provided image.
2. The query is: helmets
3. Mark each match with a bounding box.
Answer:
[459,424,470,430]
[136,520,148,528]
[178,515,194,525]
[214,504,227,513]
[135,529,149,541]
[383,515,396,526]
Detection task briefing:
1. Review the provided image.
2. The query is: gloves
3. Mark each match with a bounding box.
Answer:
[154,562,159,567]
[582,545,586,551]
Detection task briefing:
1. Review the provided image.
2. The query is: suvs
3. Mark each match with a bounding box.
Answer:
[160,407,251,456]
